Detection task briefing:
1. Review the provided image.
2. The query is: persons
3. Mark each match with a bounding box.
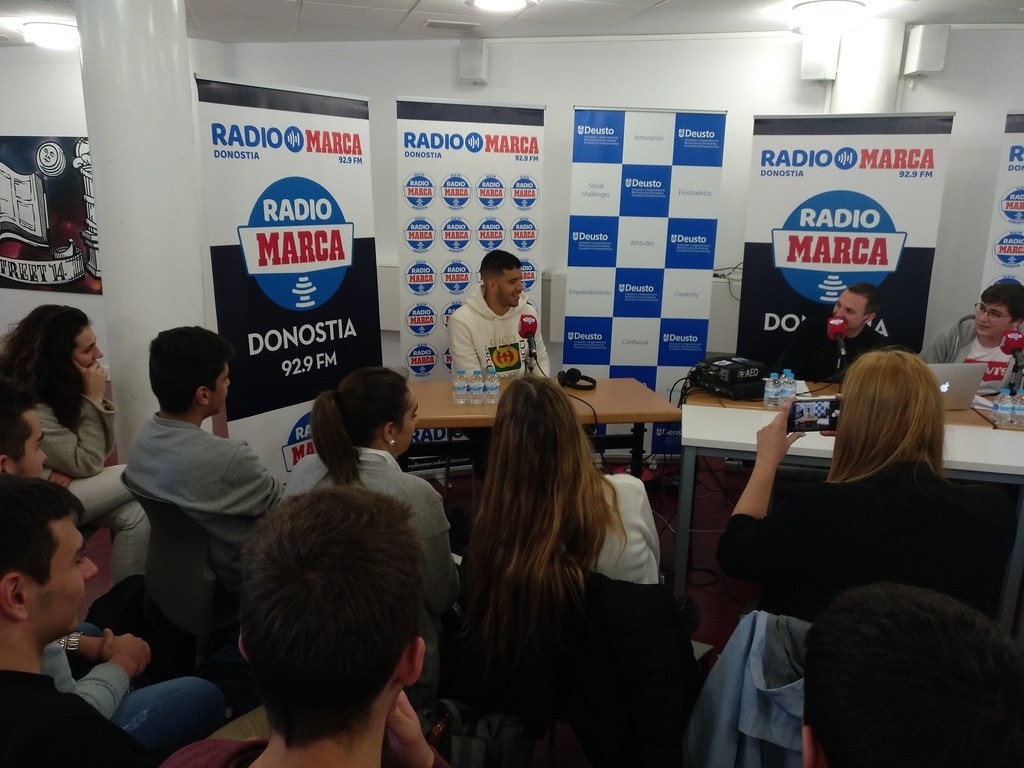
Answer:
[715,348,1019,620]
[805,408,813,428]
[771,284,888,382]
[921,282,1024,390]
[0,306,152,583]
[800,584,1024,767]
[465,378,658,676]
[125,326,285,514]
[0,379,224,768]
[446,250,550,477]
[282,366,462,618]
[158,488,452,768]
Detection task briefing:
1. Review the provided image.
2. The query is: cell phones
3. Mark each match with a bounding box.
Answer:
[787,398,841,433]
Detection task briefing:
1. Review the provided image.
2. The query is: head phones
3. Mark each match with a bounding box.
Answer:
[558,368,596,390]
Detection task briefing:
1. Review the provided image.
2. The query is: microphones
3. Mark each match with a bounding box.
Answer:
[518,314,538,361]
[998,328,1024,374]
[827,317,849,357]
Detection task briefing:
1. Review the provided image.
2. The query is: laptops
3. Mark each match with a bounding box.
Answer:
[926,362,988,411]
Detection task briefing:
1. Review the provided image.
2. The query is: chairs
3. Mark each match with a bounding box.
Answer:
[121,468,238,669]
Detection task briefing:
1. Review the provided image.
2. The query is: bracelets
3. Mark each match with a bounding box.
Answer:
[67,632,80,650]
[60,637,65,648]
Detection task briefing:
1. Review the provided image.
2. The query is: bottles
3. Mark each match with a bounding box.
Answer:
[453,370,468,404]
[468,370,484,404]
[764,373,781,409]
[485,370,501,404]
[1011,389,1024,427]
[780,369,792,381]
[779,374,796,407]
[990,389,1013,426]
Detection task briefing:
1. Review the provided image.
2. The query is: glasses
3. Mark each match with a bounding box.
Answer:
[975,303,1012,321]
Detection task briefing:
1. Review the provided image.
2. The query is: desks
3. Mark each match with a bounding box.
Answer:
[675,382,1024,642]
[399,378,682,478]
